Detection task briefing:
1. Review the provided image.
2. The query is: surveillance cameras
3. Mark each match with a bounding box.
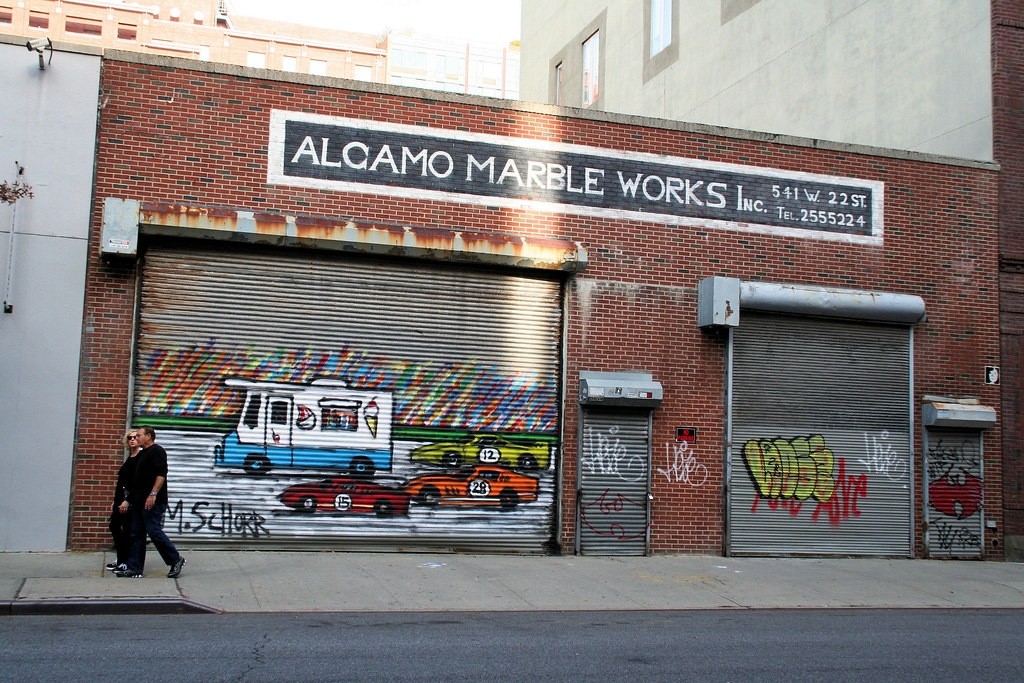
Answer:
[27,35,51,70]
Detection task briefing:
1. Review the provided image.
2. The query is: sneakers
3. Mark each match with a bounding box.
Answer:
[106,562,118,570]
[113,564,127,573]
[167,556,186,578]
[117,569,143,577]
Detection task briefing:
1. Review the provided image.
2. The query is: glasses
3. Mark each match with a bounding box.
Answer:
[136,432,149,437]
[127,435,136,440]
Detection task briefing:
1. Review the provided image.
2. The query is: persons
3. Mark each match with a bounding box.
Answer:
[104,428,142,569]
[112,425,187,578]
